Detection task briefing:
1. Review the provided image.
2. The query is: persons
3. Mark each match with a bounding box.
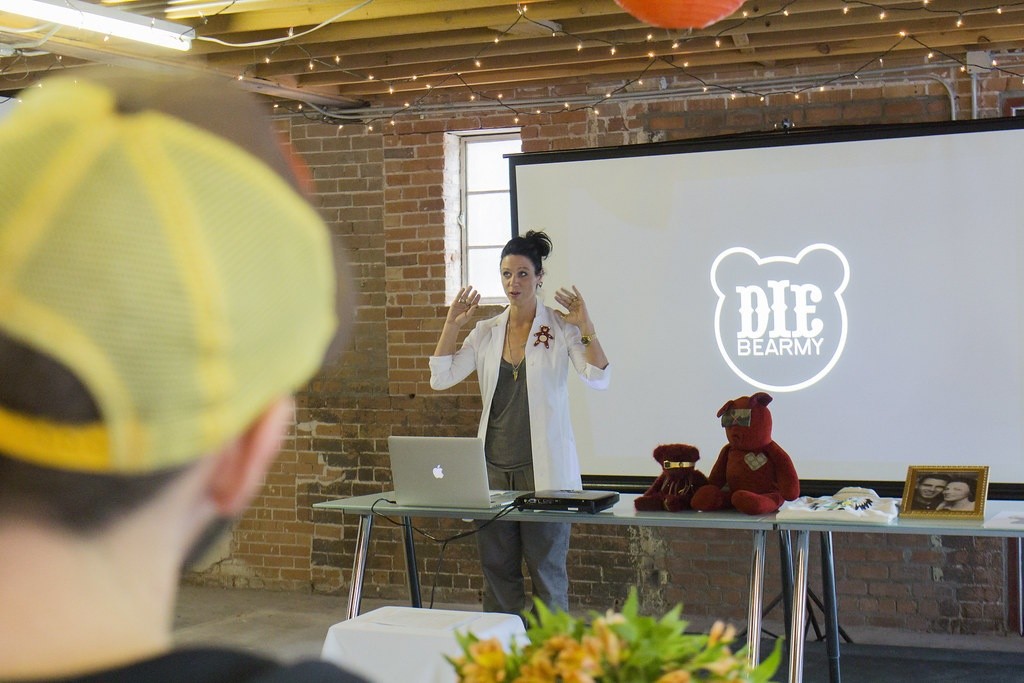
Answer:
[429,230,611,633]
[0,60,375,683]
[913,474,950,511]
[936,477,975,512]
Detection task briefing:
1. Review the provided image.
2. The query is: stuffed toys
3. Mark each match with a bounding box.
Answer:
[634,444,707,513]
[692,392,800,516]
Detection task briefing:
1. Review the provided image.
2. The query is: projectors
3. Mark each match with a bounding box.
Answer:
[517,489,620,513]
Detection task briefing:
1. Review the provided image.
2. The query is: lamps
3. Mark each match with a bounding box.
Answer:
[0,0,196,52]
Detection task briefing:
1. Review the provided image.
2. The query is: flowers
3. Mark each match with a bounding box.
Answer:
[441,585,783,682]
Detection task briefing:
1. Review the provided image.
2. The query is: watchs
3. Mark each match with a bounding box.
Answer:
[579,333,597,346]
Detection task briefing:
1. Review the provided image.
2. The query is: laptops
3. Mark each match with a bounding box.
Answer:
[388,435,534,508]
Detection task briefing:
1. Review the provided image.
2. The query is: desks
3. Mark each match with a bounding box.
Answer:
[776,498,1024,683]
[314,490,782,682]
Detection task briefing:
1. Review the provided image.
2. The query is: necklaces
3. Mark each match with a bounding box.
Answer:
[507,320,525,381]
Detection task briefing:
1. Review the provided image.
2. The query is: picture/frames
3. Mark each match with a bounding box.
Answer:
[898,466,991,521]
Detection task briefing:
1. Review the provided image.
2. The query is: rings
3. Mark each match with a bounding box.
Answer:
[560,314,565,318]
[568,305,571,310]
[570,296,577,299]
[466,299,471,304]
[461,298,465,303]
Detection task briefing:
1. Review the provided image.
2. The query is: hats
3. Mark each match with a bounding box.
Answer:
[0,54,355,474]
